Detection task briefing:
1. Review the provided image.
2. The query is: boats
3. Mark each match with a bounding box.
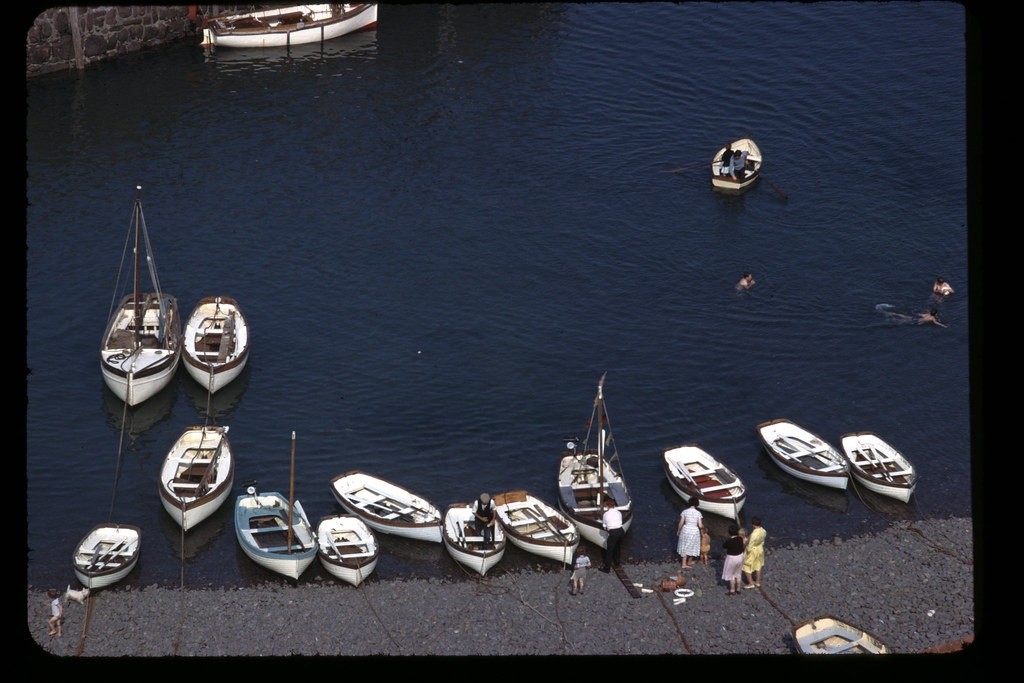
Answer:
[838,430,917,504]
[202,3,377,52]
[756,418,852,491]
[709,135,762,195]
[235,492,318,580]
[443,502,505,576]
[318,513,377,587]
[556,380,634,550]
[157,426,235,532]
[181,294,249,397]
[661,443,747,520]
[330,473,445,544]
[72,524,142,591]
[491,491,579,566]
[99,196,182,410]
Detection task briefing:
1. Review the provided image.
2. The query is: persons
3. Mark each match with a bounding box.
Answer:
[721,144,734,176]
[721,525,745,594]
[699,528,710,565]
[598,500,623,573]
[676,497,704,568]
[920,308,938,323]
[742,517,767,588]
[472,494,497,549]
[730,149,749,179]
[568,546,591,596]
[47,589,62,638]
[740,273,752,285]
[933,278,954,293]
[739,528,748,544]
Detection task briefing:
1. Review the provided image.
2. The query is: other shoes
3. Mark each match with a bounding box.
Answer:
[598,568,608,573]
[578,591,583,594]
[756,584,760,587]
[736,591,740,594]
[682,560,695,569]
[569,590,575,595]
[744,584,755,589]
[726,592,735,596]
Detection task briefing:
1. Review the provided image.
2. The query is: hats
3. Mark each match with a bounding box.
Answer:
[480,493,490,503]
